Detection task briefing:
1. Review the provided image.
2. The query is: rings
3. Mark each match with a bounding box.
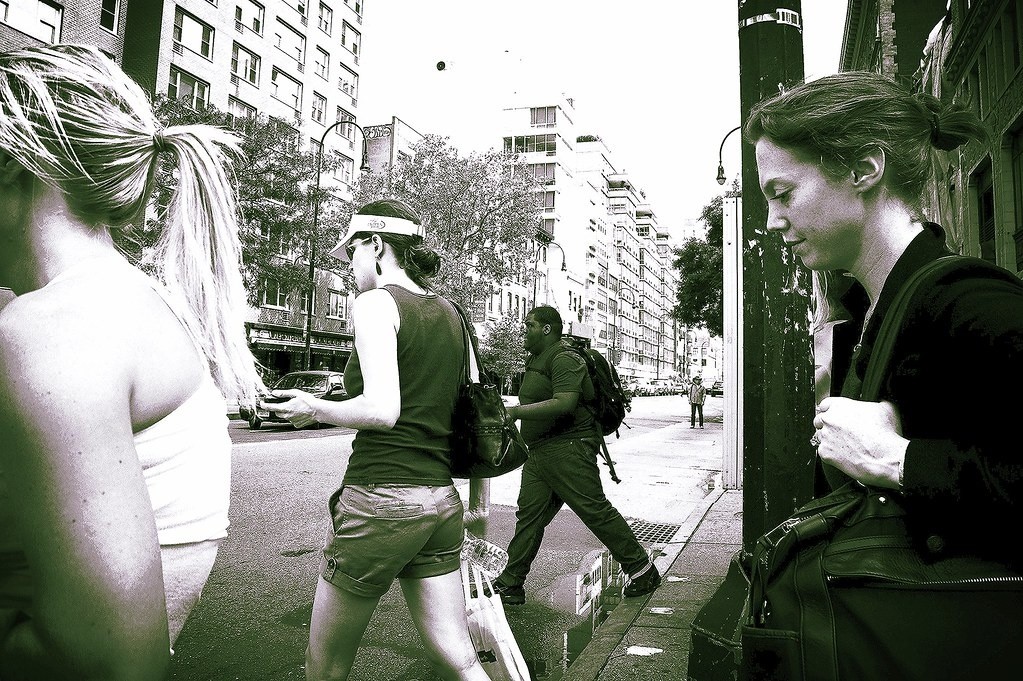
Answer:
[809,434,821,446]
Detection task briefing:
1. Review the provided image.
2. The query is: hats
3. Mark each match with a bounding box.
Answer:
[329,216,427,262]
[693,376,702,383]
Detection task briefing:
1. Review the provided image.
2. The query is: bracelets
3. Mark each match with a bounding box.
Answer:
[512,406,518,418]
[898,449,906,494]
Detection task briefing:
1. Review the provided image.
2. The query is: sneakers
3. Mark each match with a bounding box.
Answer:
[473,581,526,605]
[624,562,662,596]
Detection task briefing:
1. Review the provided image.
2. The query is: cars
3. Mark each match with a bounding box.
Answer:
[238,371,348,431]
[620,379,692,396]
[705,387,712,395]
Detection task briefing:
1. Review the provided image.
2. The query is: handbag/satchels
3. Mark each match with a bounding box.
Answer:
[460,555,531,681]
[741,256,1023,681]
[448,298,528,479]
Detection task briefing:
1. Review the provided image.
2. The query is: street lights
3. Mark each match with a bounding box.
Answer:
[304,120,372,370]
[532,241,569,308]
[613,286,637,366]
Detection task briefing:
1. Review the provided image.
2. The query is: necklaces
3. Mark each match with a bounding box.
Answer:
[853,302,877,351]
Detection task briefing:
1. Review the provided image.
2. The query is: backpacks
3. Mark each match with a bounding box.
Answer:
[545,339,630,435]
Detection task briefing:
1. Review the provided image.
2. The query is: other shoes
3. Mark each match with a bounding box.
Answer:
[690,425,694,429]
[700,426,704,429]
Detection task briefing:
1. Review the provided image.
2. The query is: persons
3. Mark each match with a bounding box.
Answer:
[261,197,491,681]
[473,308,661,605]
[745,71,1022,573]
[680,379,689,397]
[0,42,268,681]
[811,267,855,403]
[505,374,520,396]
[689,376,706,429]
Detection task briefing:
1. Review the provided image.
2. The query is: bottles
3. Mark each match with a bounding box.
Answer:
[459,530,510,579]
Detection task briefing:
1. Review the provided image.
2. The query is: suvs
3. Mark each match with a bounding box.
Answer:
[711,380,724,397]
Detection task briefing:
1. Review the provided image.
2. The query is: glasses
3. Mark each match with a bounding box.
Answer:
[345,236,371,261]
